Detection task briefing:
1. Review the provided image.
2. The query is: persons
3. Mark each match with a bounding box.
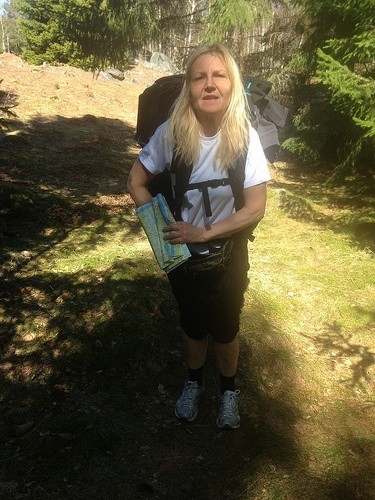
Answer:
[126,41,270,427]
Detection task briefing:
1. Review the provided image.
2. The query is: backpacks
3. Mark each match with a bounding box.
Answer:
[134,73,289,242]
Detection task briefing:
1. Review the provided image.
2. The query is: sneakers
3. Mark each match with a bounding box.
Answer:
[174,378,241,430]
